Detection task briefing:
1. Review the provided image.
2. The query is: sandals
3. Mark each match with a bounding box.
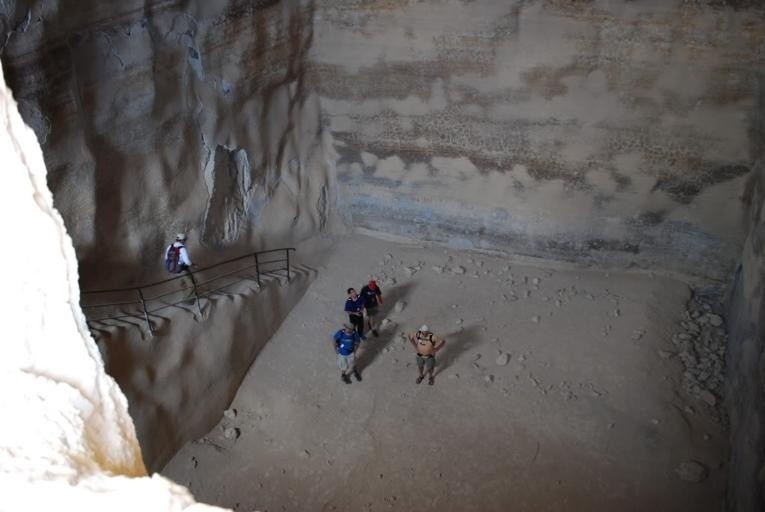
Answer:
[416,377,433,385]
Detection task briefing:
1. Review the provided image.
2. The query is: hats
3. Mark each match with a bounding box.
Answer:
[175,233,186,240]
[420,324,430,332]
[343,322,354,330]
[369,280,376,289]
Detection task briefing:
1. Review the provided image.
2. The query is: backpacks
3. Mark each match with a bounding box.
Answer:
[165,244,184,273]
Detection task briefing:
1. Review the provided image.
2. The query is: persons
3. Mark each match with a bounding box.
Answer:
[163,233,199,305]
[331,322,362,383]
[408,324,445,385]
[359,278,385,339]
[343,287,367,341]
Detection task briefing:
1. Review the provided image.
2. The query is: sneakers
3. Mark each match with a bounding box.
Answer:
[372,329,378,337]
[341,372,361,383]
